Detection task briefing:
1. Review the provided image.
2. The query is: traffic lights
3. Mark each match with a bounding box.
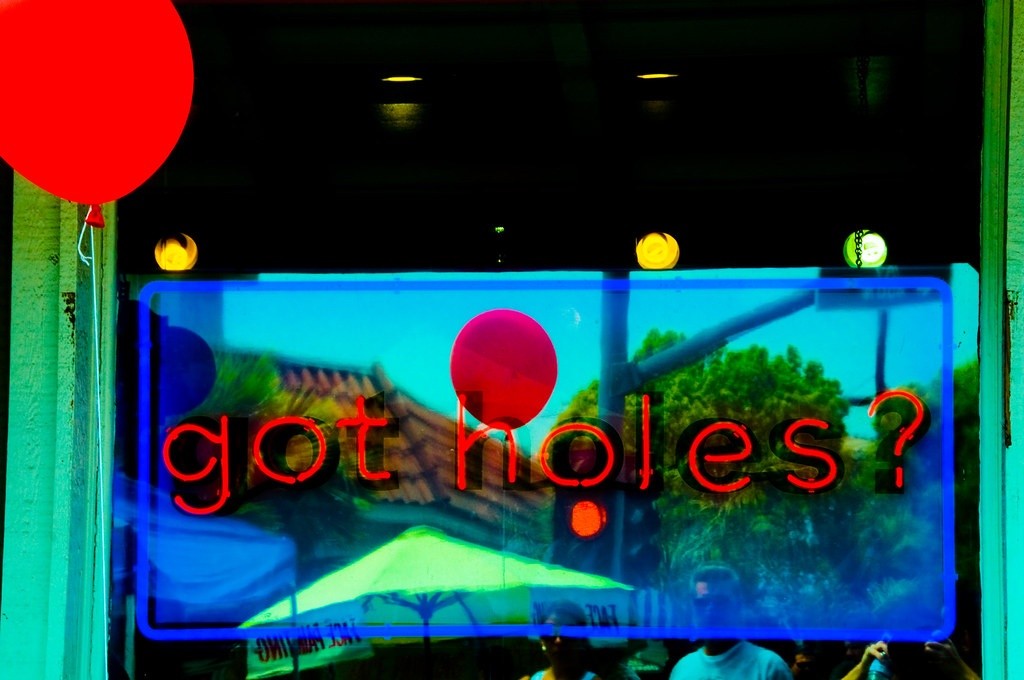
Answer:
[164,410,342,500]
[541,429,612,595]
[613,454,662,592]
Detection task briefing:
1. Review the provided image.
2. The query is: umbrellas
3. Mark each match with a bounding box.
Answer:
[234,522,637,680]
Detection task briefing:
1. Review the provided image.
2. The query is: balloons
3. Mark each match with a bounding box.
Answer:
[0,0,194,229]
[449,309,558,428]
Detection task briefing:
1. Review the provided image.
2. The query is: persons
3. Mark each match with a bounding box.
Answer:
[669,566,793,680]
[792,600,981,680]
[520,600,603,680]
